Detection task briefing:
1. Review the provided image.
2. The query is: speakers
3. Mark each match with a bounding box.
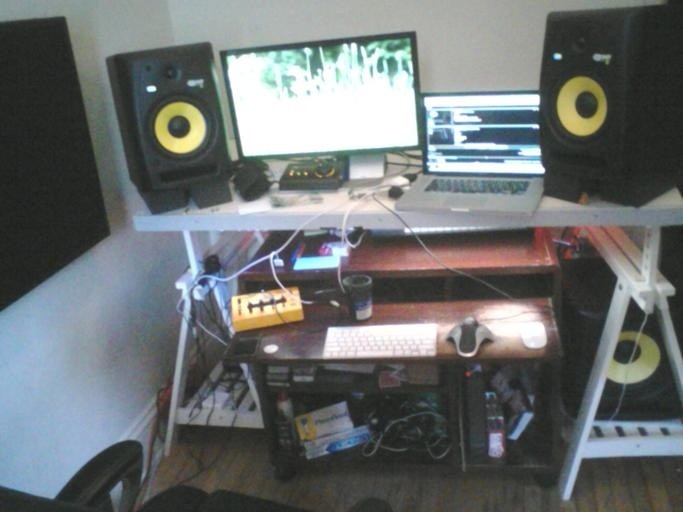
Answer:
[539,2,683,209]
[559,255,682,422]
[105,41,234,215]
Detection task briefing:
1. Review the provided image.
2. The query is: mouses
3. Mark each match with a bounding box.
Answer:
[520,321,547,349]
[445,317,496,358]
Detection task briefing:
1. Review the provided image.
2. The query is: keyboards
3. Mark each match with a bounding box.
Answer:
[322,323,438,360]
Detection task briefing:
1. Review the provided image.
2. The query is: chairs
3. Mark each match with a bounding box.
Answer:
[0,438,394,512]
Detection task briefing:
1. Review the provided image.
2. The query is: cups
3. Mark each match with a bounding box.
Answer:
[343,276,373,322]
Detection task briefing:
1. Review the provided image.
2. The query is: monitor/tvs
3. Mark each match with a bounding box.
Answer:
[1,15,111,312]
[219,30,423,161]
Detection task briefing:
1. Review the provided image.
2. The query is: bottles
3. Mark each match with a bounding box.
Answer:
[276,391,300,447]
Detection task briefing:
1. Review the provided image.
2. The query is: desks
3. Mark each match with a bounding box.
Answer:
[131,168,683,502]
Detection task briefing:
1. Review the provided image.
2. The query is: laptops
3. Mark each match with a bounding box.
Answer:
[393,88,545,217]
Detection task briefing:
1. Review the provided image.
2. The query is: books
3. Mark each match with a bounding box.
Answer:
[486,367,534,458]
